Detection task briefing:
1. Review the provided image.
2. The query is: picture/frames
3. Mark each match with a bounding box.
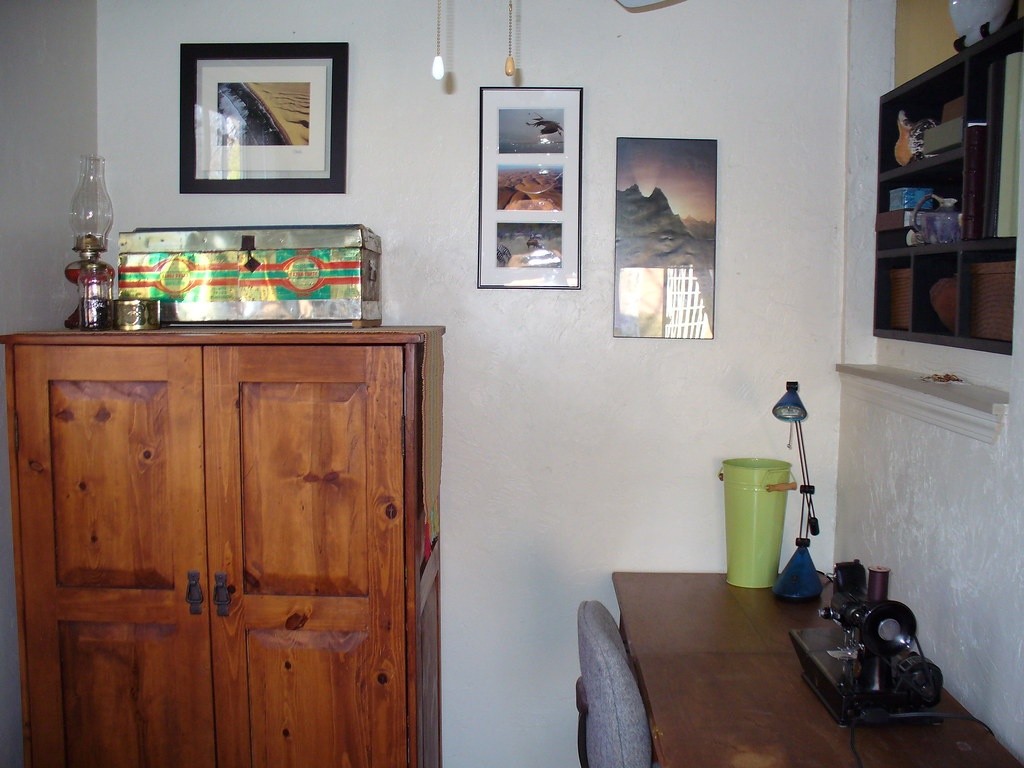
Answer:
[477,87,584,290]
[179,42,346,194]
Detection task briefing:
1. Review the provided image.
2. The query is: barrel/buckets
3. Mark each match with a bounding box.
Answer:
[716,458,797,589]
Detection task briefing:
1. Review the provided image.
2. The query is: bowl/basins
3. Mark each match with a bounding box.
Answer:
[109,299,161,331]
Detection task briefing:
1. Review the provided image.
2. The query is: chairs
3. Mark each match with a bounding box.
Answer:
[577,600,654,768]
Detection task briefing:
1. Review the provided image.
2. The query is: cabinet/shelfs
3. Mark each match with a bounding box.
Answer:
[0,324,448,768]
[872,17,1024,358]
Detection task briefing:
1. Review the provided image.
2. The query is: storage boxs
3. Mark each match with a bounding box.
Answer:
[118,224,384,322]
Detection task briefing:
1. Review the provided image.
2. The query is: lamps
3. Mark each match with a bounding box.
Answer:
[772,381,823,601]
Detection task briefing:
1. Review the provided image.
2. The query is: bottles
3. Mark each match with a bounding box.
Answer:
[77,264,114,331]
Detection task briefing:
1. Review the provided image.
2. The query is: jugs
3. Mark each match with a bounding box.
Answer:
[910,194,960,244]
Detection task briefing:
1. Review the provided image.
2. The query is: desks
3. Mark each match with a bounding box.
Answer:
[613,571,1024,768]
